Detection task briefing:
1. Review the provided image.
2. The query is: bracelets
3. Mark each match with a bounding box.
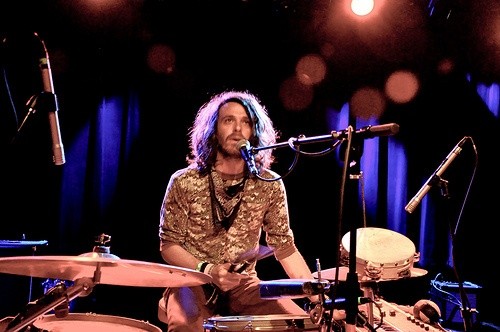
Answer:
[197,261,216,276]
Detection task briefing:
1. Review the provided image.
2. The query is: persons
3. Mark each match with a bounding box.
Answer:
[159,92,331,332]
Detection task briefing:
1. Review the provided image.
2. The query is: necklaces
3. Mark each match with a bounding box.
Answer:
[207,169,249,237]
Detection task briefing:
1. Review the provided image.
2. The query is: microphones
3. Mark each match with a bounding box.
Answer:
[237,138,258,175]
[305,296,371,310]
[405,137,468,213]
[39,41,65,164]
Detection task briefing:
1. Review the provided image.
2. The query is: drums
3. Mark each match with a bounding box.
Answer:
[25,313,163,332]
[205,314,326,332]
[339,227,421,281]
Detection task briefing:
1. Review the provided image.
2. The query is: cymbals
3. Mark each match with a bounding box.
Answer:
[397,262,412,278]
[413,252,421,263]
[0,251,213,288]
[340,255,349,268]
[363,267,382,282]
[312,266,428,282]
